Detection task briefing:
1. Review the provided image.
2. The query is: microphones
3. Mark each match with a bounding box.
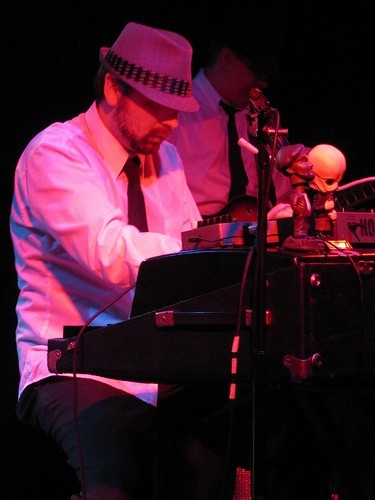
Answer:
[249,88,278,129]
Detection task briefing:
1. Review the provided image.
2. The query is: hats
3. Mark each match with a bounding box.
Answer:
[227,23,297,81]
[100,22,200,113]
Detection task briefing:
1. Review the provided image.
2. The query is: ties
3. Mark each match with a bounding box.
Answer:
[124,158,148,232]
[219,100,248,202]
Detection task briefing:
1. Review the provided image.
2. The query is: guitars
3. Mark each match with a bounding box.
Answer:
[197,181,375,229]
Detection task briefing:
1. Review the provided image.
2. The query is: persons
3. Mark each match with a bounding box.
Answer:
[10,21,205,499]
[167,20,311,217]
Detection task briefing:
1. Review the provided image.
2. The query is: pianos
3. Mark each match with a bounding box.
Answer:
[181,209,375,250]
[48,248,375,500]
